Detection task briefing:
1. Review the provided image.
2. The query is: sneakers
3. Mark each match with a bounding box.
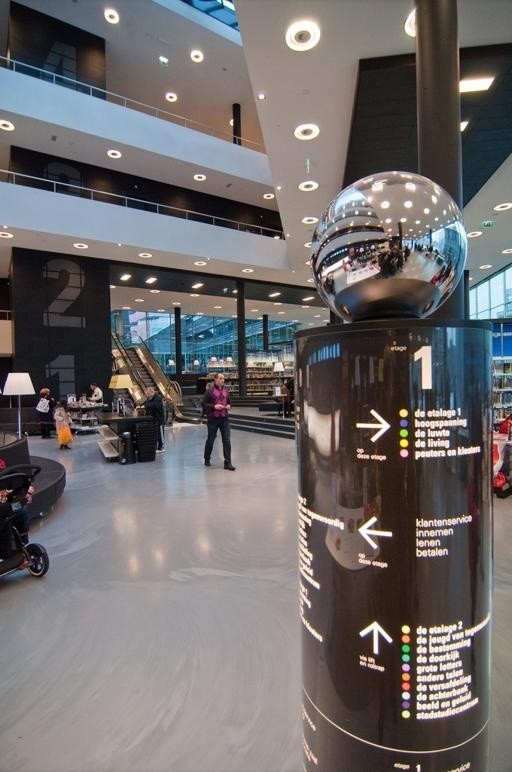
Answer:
[205,457,235,471]
[60,444,72,450]
[156,448,165,453]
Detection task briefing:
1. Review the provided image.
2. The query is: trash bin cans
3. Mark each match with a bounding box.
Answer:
[118,431,136,465]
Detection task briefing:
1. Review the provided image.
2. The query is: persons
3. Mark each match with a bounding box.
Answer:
[38,387,57,439]
[53,401,74,451]
[367,346,422,675]
[88,383,104,424]
[321,235,456,307]
[200,373,236,472]
[140,386,166,453]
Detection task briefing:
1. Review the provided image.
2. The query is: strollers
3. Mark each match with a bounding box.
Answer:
[0,463,49,577]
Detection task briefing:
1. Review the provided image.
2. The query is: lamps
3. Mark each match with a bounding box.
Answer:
[3,372,36,438]
[273,363,284,386]
[108,374,134,417]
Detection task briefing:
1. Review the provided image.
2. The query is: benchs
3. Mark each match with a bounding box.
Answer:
[17,456,66,515]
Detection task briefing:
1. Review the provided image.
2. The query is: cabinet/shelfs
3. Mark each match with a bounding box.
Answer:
[493,373,512,424]
[64,404,108,435]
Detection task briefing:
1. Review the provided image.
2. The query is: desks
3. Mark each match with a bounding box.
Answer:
[272,394,294,416]
[94,412,156,464]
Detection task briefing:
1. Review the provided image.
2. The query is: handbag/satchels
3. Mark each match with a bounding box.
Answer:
[58,426,73,443]
[36,397,50,413]
